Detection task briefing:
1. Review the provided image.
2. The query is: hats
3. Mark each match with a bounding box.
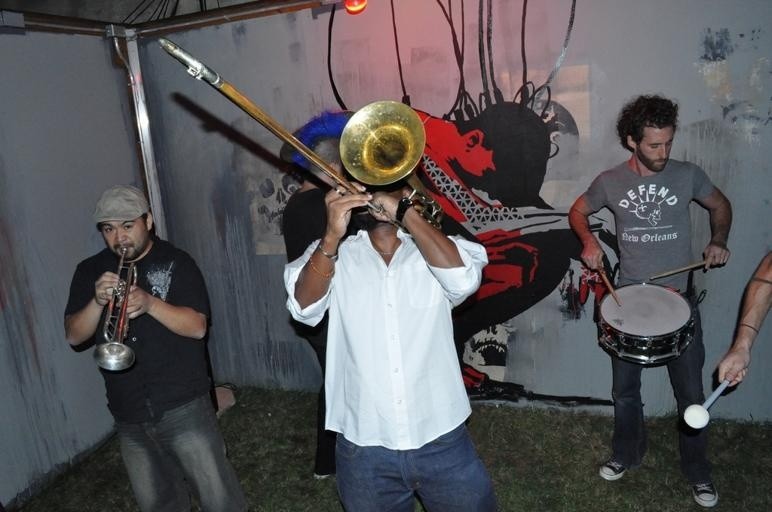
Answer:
[93,184,149,223]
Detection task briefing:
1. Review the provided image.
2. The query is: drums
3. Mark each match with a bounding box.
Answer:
[598,282,698,367]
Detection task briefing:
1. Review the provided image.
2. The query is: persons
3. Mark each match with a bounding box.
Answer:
[282,173,500,512]
[568,92,734,510]
[282,135,364,482]
[59,183,254,512]
[716,251,772,390]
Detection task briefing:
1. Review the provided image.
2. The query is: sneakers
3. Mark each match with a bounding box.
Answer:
[599,454,627,481]
[691,480,718,507]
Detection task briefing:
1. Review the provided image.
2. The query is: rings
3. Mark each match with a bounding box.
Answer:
[333,184,346,198]
[707,255,713,258]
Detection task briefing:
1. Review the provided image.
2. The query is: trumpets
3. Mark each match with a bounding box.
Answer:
[92,247,140,373]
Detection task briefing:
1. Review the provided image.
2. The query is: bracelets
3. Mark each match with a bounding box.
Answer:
[316,241,338,262]
[737,324,760,338]
[308,256,335,279]
[395,195,415,224]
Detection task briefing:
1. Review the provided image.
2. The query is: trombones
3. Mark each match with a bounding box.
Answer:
[155,37,444,238]
